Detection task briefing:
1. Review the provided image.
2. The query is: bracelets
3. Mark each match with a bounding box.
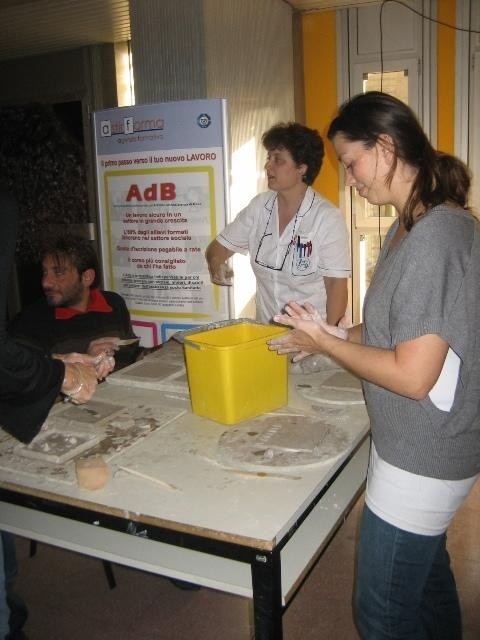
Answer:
[61,364,84,399]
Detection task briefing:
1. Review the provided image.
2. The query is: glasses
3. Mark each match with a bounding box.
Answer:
[253,232,294,272]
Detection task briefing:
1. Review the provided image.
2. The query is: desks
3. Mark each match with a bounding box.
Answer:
[0,328,373,639]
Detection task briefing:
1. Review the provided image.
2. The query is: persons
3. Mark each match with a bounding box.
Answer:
[6,229,205,592]
[265,88,480,640]
[204,118,354,332]
[1,330,120,638]
